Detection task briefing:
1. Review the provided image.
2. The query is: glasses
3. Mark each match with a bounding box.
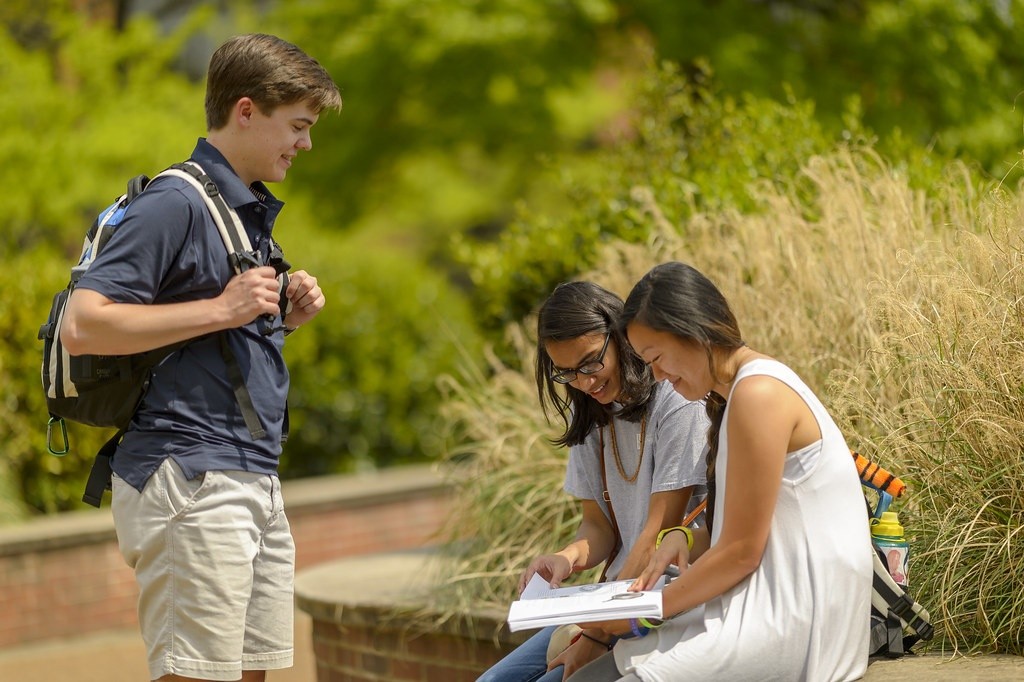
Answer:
[548,332,612,385]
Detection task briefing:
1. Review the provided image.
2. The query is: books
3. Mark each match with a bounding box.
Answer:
[507,570,670,633]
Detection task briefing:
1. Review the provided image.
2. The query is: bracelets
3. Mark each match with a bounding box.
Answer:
[675,523,676,524]
[579,632,610,650]
[629,618,668,637]
[657,525,694,551]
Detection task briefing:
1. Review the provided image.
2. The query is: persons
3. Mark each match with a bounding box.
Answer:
[59,32,345,682]
[474,262,872,682]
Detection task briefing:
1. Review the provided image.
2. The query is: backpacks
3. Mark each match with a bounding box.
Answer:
[38,162,294,428]
[870,539,933,657]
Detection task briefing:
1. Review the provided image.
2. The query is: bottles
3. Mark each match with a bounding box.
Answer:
[870,512,909,587]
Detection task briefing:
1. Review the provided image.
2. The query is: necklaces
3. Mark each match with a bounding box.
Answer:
[607,411,647,482]
